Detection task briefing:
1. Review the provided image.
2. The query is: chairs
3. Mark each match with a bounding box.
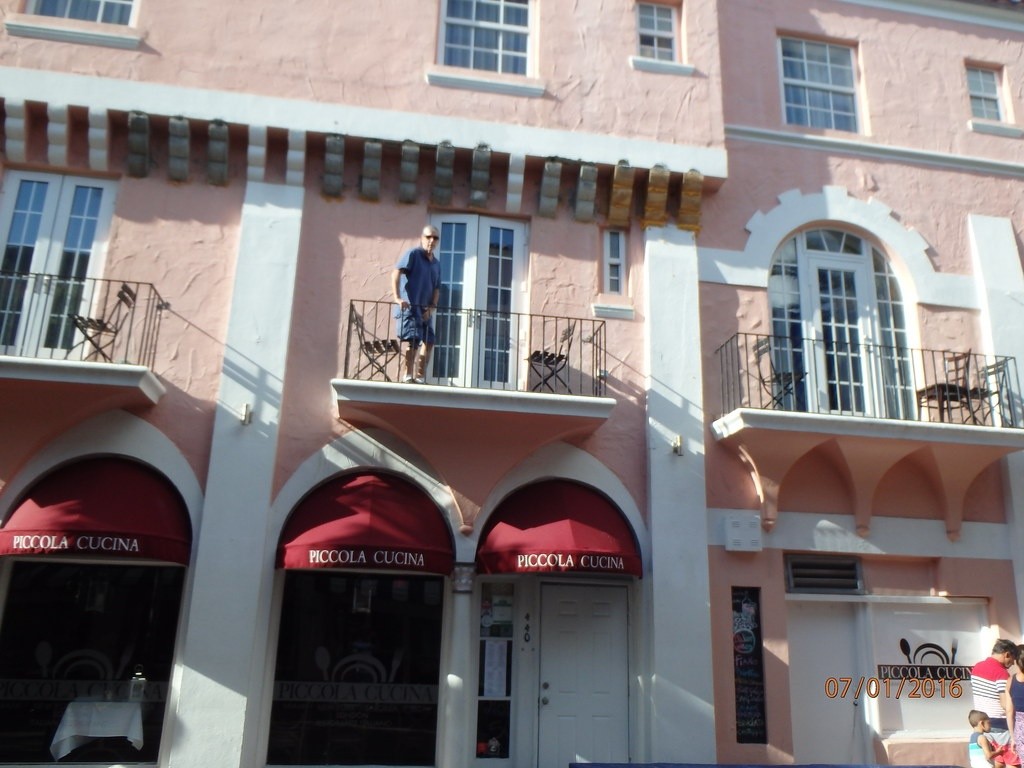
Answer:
[963,357,1013,428]
[916,349,978,426]
[351,303,400,383]
[524,320,577,395]
[63,281,137,363]
[752,336,808,411]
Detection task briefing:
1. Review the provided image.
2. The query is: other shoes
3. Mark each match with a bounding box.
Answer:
[414,379,428,385]
[401,378,418,384]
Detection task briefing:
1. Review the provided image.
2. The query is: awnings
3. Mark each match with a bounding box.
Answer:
[477,481,642,580]
[277,470,456,574]
[0,459,190,567]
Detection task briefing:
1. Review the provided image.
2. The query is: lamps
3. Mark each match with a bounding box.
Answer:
[241,403,253,424]
[672,435,685,456]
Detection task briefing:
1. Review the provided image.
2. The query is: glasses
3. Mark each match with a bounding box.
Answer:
[423,234,439,241]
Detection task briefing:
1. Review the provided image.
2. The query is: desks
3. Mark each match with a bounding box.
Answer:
[50,701,145,762]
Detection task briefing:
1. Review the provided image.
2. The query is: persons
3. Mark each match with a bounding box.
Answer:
[968,710,1006,768]
[1005,645,1024,768]
[391,225,442,384]
[971,639,1022,768]
